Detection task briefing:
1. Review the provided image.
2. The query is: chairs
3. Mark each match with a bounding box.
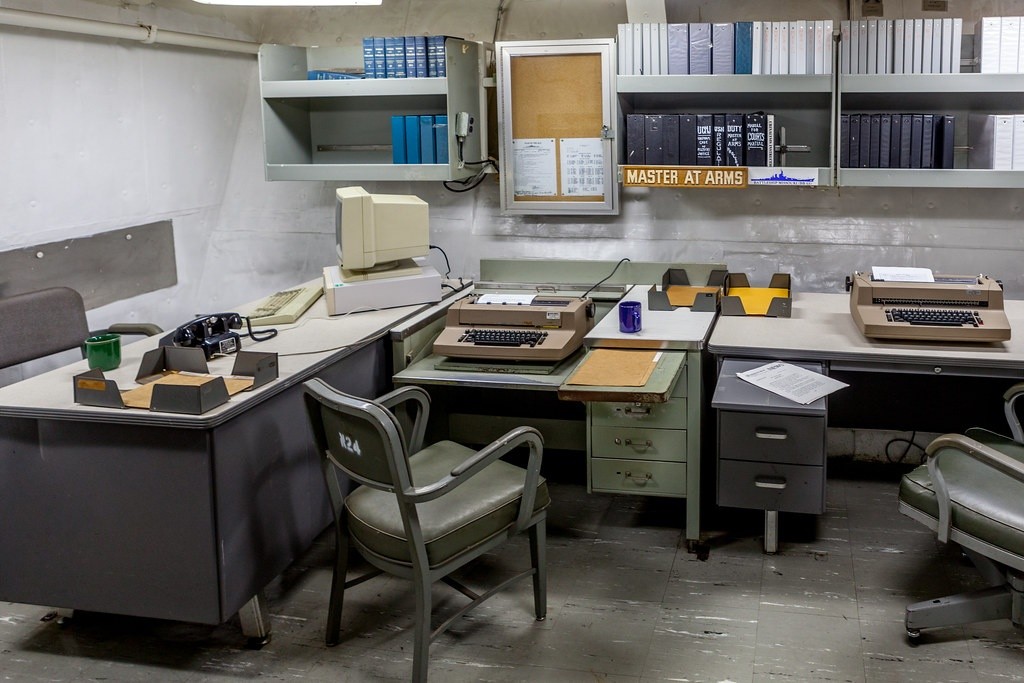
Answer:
[295,375,557,682]
[898,381,1024,649]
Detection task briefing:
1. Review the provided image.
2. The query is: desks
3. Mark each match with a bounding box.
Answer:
[0,262,1024,620]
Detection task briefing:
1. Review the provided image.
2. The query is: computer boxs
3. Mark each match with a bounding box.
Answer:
[323,257,442,316]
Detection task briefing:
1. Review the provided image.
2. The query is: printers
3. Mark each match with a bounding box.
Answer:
[845,270,1011,341]
[433,295,595,362]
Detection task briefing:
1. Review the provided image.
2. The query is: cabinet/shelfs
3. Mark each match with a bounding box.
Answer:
[254,32,491,189]
[610,15,1024,192]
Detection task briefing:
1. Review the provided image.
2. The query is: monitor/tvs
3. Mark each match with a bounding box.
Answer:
[334,186,429,272]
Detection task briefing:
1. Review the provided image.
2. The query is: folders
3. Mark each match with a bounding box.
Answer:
[304,13,1024,172]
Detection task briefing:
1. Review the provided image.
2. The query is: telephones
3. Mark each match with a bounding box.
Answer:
[173,311,243,360]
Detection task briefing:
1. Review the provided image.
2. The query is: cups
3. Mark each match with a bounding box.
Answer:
[619,301,642,335]
[83,334,121,372]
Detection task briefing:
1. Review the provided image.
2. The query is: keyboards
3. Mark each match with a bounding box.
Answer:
[240,287,323,327]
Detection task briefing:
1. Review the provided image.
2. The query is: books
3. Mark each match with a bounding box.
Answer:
[306,35,464,78]
[614,13,1022,74]
[625,109,1023,170]
[390,114,449,166]
